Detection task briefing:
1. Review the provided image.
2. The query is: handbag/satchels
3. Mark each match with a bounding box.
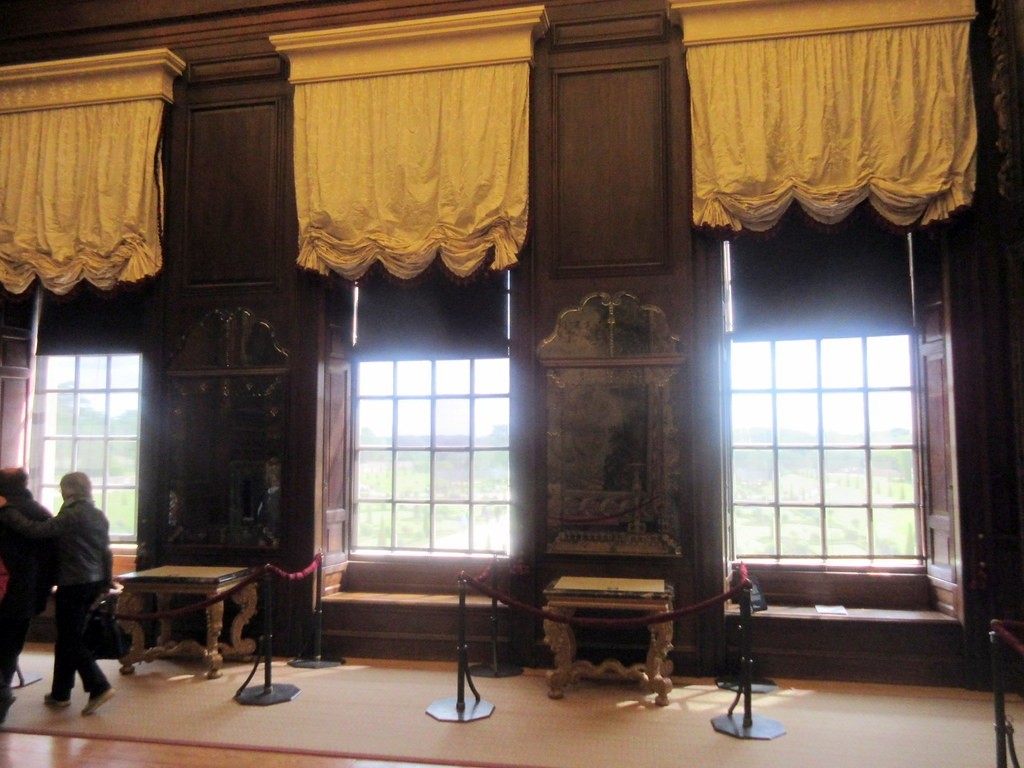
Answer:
[81,597,130,662]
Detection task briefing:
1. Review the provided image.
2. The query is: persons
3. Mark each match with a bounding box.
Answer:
[0,467,54,724]
[0,472,116,716]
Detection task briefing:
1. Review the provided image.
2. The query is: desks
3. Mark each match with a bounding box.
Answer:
[541,575,677,707]
[114,562,260,681]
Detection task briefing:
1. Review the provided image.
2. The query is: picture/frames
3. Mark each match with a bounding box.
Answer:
[158,366,299,560]
[538,354,689,560]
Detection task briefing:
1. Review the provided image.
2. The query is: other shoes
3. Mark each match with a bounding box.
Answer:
[44,690,72,707]
[81,686,117,712]
